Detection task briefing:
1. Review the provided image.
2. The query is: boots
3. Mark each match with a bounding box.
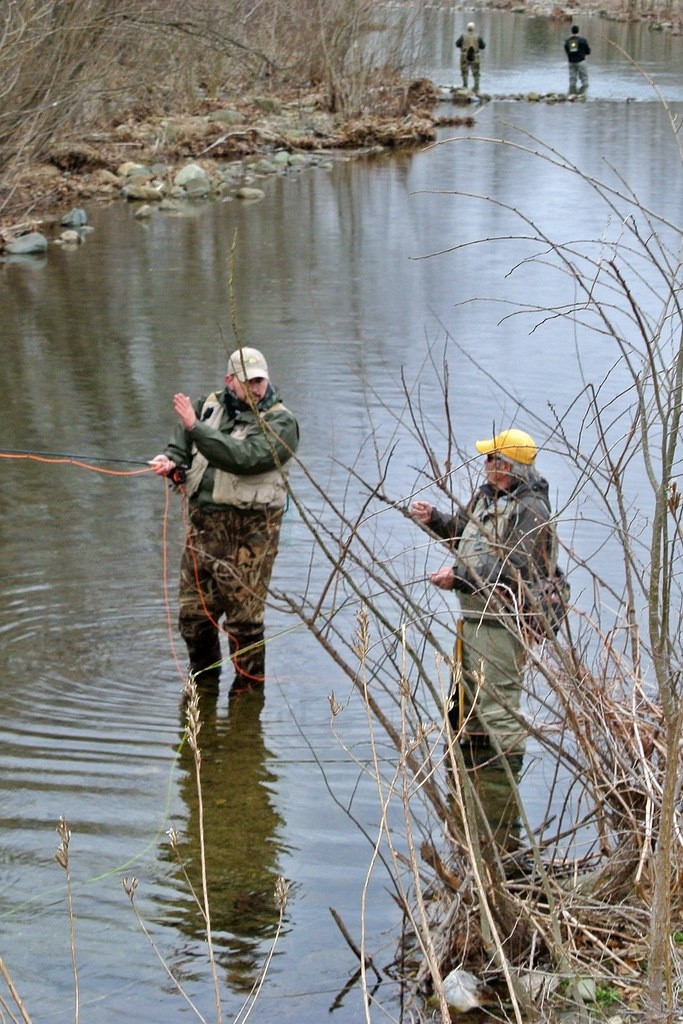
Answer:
[223,623,265,697]
[177,618,222,697]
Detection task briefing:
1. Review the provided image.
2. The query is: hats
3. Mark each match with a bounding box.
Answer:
[467,22,476,30]
[475,429,537,466]
[571,25,579,34]
[227,346,269,382]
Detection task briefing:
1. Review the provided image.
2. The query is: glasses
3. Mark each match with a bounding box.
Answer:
[487,455,500,462]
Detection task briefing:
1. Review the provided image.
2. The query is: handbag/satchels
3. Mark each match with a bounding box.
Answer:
[519,576,571,644]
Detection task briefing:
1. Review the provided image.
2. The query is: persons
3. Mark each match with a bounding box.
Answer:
[411,428,557,770]
[150,347,300,690]
[564,26,591,86]
[456,21,486,74]
